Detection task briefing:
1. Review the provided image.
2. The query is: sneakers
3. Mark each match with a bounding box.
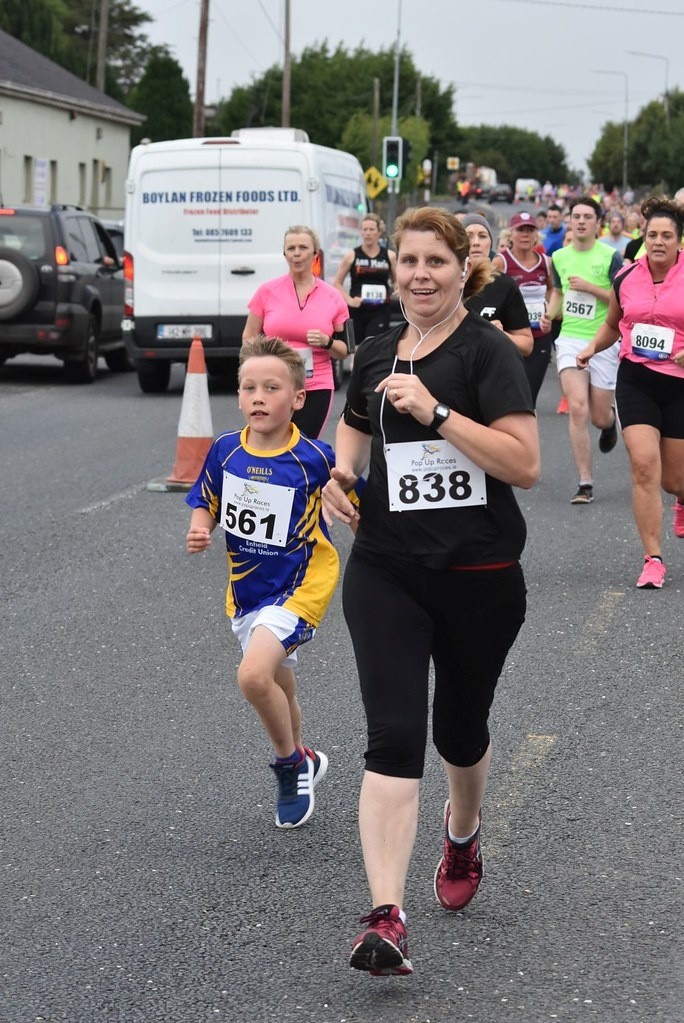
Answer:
[269,744,314,828]
[350,904,413,976]
[636,554,666,588]
[434,800,484,910]
[570,484,593,503]
[599,405,618,453]
[303,746,328,788]
[670,497,684,538]
[556,397,569,414]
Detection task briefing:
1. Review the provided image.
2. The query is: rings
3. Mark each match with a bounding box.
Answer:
[394,388,398,399]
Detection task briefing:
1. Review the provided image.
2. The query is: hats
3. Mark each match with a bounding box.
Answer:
[509,213,538,230]
[460,214,493,249]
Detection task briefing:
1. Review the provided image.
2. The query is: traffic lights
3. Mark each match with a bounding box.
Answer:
[382,135,403,180]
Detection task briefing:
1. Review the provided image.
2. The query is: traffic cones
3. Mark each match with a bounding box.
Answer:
[535,194,540,207]
[515,192,519,203]
[541,192,545,204]
[548,195,553,207]
[146,335,215,494]
[525,194,529,201]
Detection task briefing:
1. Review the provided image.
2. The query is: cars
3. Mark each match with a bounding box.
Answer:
[488,184,513,204]
[475,184,494,201]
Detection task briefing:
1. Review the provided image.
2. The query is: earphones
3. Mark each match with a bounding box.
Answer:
[462,257,469,277]
[314,251,316,254]
[283,252,285,256]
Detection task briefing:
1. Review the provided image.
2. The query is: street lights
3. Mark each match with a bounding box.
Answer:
[600,70,627,193]
[629,50,669,116]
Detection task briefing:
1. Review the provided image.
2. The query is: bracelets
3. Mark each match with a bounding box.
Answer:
[321,336,333,350]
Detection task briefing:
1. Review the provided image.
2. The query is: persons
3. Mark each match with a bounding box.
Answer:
[318,205,541,978]
[537,198,617,505]
[334,214,399,352]
[456,212,534,359]
[575,197,684,590]
[492,211,555,412]
[241,224,356,444]
[448,172,684,257]
[186,337,367,829]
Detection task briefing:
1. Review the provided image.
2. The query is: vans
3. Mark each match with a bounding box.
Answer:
[123,128,369,395]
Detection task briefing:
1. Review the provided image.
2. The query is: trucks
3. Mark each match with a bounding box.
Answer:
[516,179,539,198]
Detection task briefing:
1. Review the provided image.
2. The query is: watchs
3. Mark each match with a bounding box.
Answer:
[429,402,451,432]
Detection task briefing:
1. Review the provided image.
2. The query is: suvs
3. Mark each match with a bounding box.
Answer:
[0,202,137,385]
